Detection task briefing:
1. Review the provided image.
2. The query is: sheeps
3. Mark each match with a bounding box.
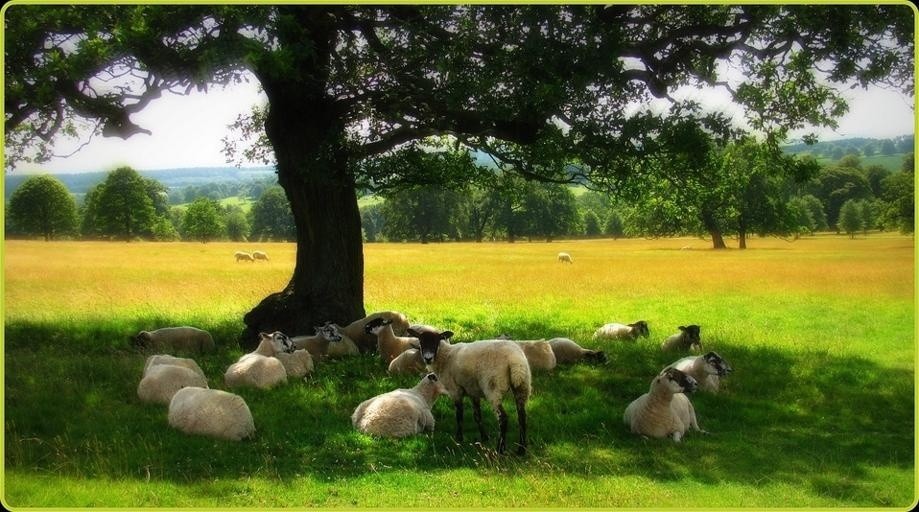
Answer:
[559,252,573,266]
[664,349,732,395]
[254,249,269,260]
[342,307,607,455]
[621,367,699,440]
[234,251,255,264]
[662,322,703,350]
[130,325,215,354]
[135,352,208,404]
[226,316,343,390]
[590,316,650,348]
[167,386,266,443]
